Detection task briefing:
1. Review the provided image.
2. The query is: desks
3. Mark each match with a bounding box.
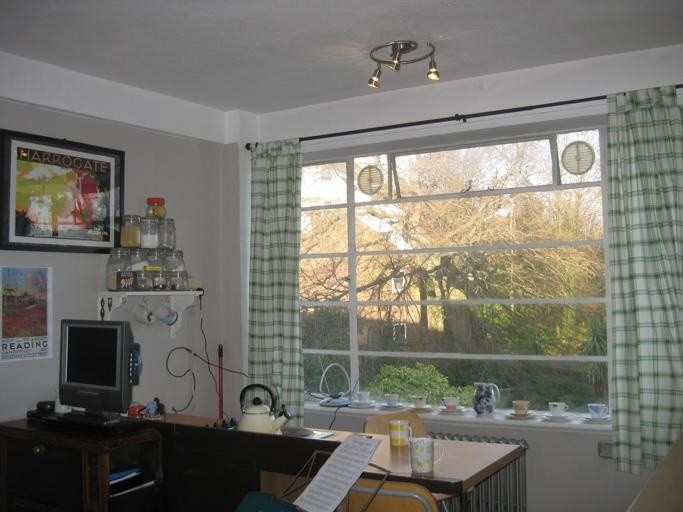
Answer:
[159,411,525,497]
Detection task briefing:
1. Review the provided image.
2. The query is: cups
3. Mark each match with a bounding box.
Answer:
[413,396,428,407]
[357,392,371,402]
[512,401,529,415]
[444,397,459,409]
[588,404,608,418]
[549,402,569,416]
[409,438,445,476]
[385,393,398,406]
[389,419,412,446]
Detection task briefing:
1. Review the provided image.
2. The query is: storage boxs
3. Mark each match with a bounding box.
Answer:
[0,426,163,511]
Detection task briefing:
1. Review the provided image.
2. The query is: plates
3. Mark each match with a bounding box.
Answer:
[380,404,405,410]
[504,411,535,419]
[408,405,432,412]
[348,400,376,408]
[439,407,464,412]
[581,418,611,423]
[543,414,573,422]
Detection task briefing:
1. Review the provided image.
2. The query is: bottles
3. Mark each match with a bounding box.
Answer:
[169,272,190,291]
[146,250,168,272]
[145,197,167,219]
[153,272,172,291]
[130,248,150,272]
[120,215,142,248]
[105,248,133,291]
[165,250,187,272]
[158,218,177,251]
[140,218,158,249]
[137,271,155,290]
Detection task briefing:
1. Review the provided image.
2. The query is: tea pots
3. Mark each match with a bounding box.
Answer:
[236,384,292,435]
[472,383,500,416]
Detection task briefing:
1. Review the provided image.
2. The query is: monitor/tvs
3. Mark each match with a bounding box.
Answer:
[58,318,132,425]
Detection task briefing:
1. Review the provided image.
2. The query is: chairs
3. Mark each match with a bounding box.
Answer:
[232,451,389,512]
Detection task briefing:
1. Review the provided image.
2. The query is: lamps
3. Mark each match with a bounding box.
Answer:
[367,40,439,89]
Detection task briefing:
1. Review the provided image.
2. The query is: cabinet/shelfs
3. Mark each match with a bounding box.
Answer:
[98,290,204,339]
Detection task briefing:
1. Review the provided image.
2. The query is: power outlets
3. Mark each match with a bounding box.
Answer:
[598,440,616,460]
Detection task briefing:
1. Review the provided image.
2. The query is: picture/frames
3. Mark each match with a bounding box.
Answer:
[0,129,125,255]
